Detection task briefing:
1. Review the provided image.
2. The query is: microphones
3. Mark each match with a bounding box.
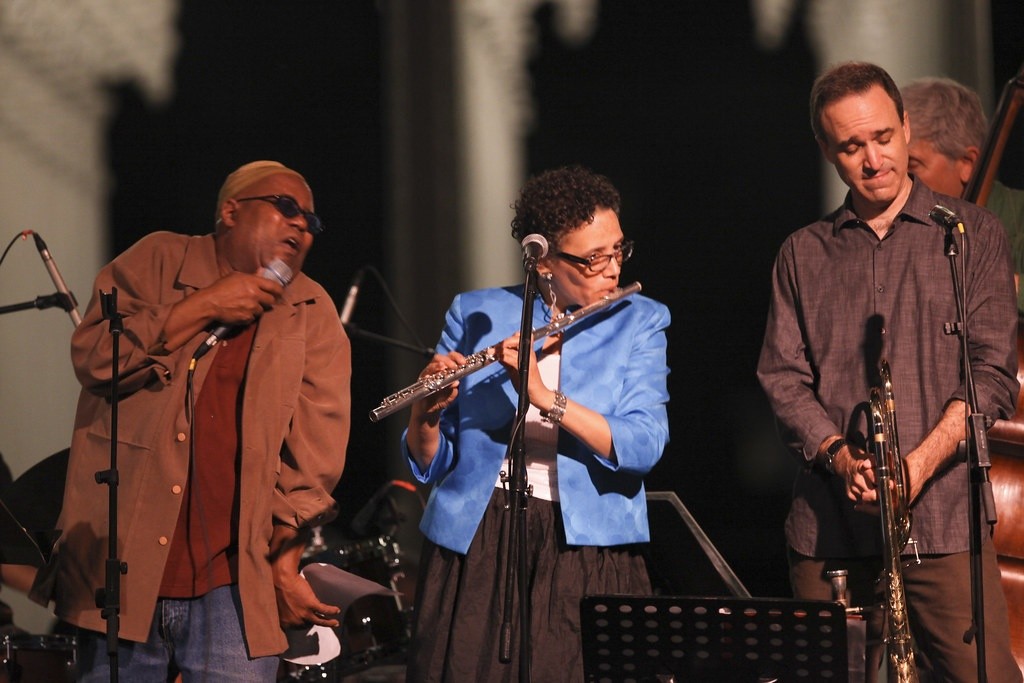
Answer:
[191,260,294,361]
[340,267,366,327]
[31,232,82,328]
[928,205,964,234]
[521,234,549,273]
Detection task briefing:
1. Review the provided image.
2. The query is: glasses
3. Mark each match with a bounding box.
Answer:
[555,239,635,273]
[236,195,324,234]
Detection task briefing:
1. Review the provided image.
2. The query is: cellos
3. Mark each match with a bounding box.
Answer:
[962,66,1024,682]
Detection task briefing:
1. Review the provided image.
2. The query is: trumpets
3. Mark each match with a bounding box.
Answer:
[869,360,921,683]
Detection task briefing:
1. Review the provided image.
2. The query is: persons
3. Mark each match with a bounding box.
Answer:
[400,165,671,683]
[53,161,351,683]
[749,62,1024,683]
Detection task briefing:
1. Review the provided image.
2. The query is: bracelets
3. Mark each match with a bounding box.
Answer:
[540,389,566,429]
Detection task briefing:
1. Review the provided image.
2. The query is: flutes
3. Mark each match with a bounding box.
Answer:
[369,282,644,423]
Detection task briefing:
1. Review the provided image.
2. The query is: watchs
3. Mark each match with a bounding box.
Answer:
[821,437,847,476]
